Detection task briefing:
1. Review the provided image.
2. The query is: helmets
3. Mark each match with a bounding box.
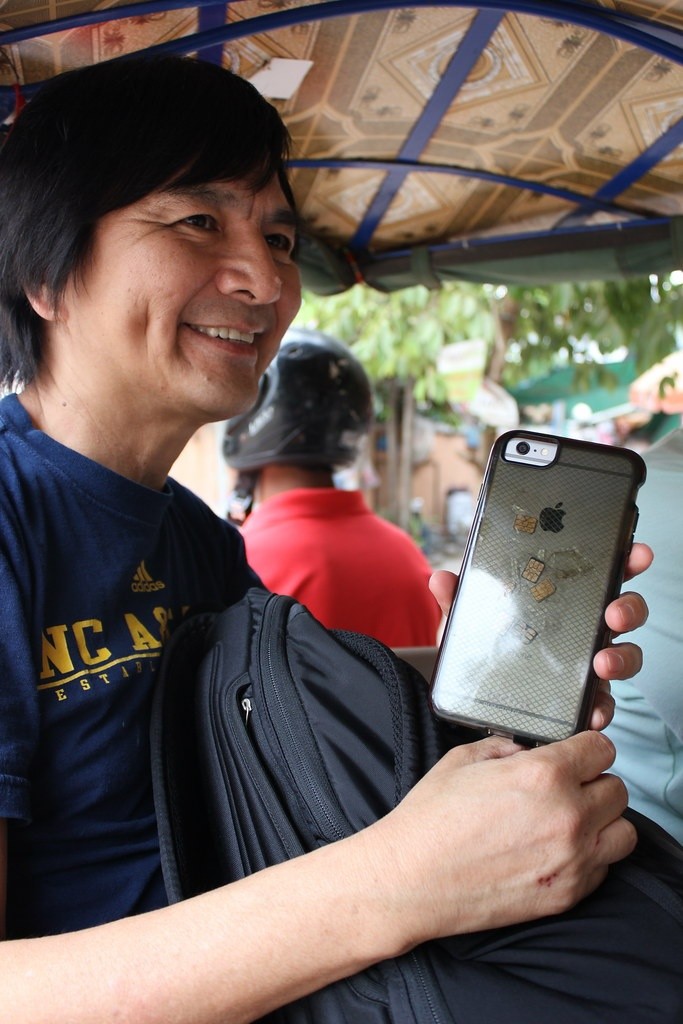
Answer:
[221,326,374,470]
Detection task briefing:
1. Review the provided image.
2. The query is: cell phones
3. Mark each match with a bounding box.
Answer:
[425,429,648,743]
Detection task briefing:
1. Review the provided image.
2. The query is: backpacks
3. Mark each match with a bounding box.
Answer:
[149,583,683,1024]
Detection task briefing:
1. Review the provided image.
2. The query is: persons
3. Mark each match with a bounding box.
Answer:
[0,54,683,1024]
